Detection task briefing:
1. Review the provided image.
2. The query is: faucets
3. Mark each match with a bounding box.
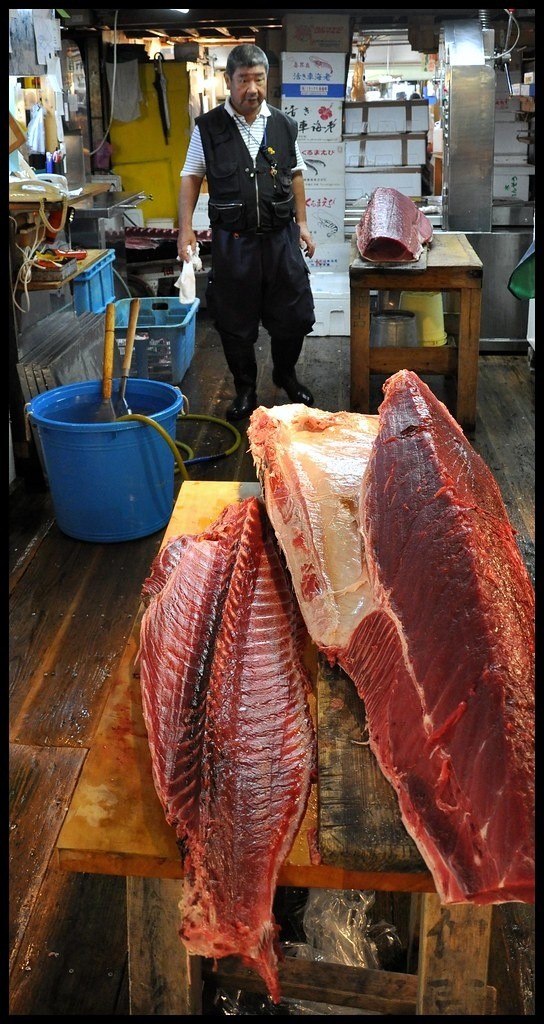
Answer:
[148,192,155,202]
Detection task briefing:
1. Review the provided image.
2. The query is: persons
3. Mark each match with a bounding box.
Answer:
[177,45,316,420]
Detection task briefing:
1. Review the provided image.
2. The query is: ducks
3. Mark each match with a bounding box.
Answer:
[350,62,366,101]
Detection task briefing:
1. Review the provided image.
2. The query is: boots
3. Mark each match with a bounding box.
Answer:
[271,335,315,407]
[225,343,257,420]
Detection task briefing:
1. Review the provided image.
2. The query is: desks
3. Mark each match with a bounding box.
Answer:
[54,476,535,1015]
[349,234,482,436]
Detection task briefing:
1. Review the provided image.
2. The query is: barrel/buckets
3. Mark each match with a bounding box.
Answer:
[23,379,189,544]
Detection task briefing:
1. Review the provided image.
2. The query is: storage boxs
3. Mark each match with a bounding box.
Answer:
[174,42,205,61]
[147,217,178,229]
[293,190,344,241]
[343,98,430,133]
[524,71,535,84]
[306,274,350,337]
[345,165,421,200]
[282,51,346,100]
[512,83,524,95]
[103,297,202,385]
[71,248,117,317]
[297,142,345,189]
[300,240,351,272]
[342,132,426,167]
[281,99,342,142]
[521,84,535,96]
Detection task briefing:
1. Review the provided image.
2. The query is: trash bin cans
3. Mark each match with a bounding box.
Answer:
[370,308,418,347]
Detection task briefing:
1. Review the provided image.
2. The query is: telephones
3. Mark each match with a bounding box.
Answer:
[9,171,69,203]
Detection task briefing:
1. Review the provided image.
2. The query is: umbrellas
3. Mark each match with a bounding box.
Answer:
[153,52,172,146]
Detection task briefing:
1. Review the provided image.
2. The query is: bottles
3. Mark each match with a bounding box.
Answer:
[46,147,64,175]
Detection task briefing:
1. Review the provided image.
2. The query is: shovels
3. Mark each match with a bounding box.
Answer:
[95,303,116,423]
[114,300,140,418]
